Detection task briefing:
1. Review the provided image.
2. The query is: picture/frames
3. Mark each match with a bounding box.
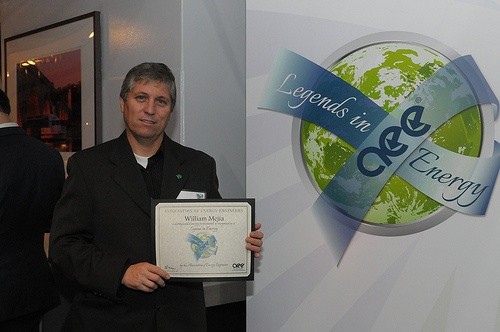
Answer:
[151,198,254,281]
[4,10,103,179]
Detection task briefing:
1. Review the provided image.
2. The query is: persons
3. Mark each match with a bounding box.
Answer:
[49,62,263,332]
[0,90,65,332]
